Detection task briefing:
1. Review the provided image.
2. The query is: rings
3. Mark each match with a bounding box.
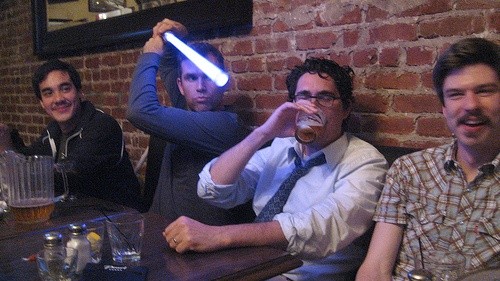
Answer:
[172,238,177,244]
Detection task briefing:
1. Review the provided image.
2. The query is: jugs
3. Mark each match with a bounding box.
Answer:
[5,150,70,224]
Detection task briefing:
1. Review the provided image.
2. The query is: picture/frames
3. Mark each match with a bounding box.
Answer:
[88,0,126,12]
[31,0,254,60]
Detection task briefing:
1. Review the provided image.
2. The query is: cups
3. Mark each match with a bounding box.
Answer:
[79,220,105,264]
[35,246,79,281]
[294,104,327,144]
[106,213,145,266]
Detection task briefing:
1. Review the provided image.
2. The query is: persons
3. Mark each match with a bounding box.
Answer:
[126,18,246,226]
[162,56,390,281]
[356,38,500,281]
[0,60,143,203]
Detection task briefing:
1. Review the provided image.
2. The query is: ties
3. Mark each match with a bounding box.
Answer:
[254,153,326,222]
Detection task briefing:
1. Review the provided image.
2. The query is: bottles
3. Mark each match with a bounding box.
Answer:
[42,231,64,281]
[65,222,92,281]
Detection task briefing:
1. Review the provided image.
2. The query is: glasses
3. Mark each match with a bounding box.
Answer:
[292,94,341,108]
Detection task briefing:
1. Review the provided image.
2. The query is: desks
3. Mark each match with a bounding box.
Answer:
[0,191,304,281]
